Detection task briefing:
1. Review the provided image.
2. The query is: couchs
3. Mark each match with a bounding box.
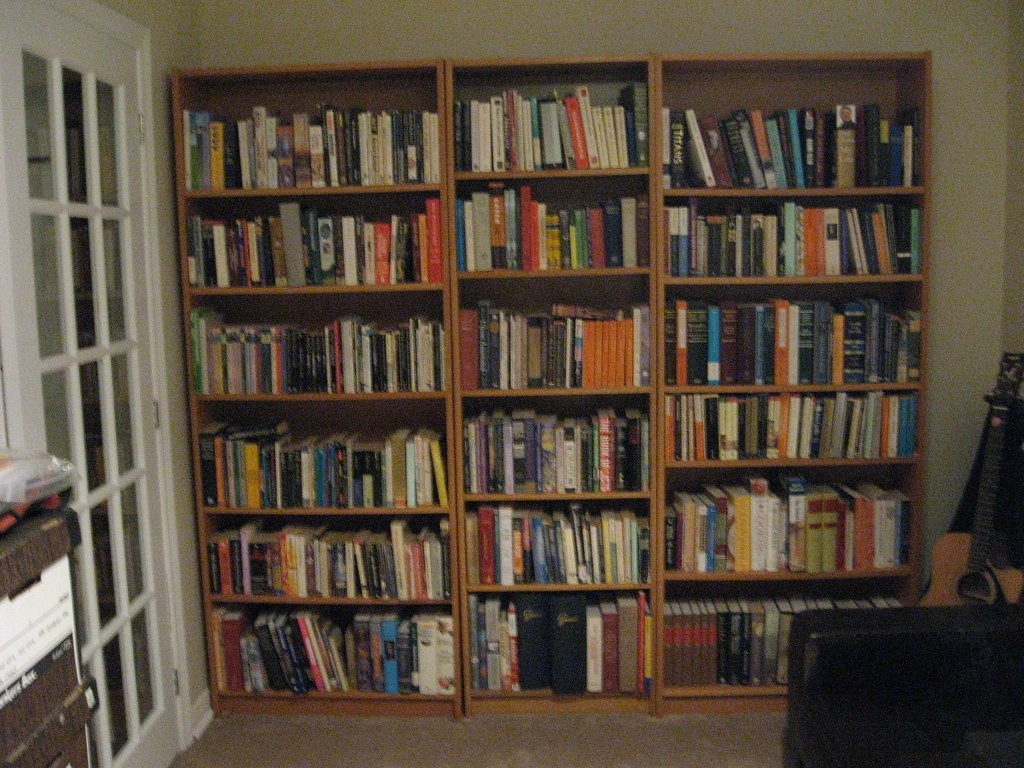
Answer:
[783,605,1024,768]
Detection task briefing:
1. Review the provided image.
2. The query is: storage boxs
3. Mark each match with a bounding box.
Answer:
[0,503,99,768]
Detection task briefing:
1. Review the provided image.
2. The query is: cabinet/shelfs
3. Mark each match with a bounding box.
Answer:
[171,51,931,716]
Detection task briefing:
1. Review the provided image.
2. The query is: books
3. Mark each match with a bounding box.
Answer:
[184,84,922,694]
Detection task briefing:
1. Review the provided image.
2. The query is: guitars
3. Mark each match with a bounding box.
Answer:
[912,350,1024,609]
